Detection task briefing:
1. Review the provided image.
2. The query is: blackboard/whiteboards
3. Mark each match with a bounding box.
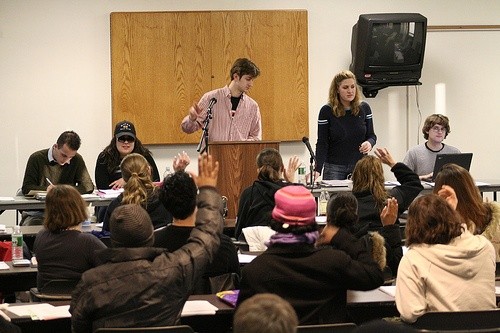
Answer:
[407,24,500,184]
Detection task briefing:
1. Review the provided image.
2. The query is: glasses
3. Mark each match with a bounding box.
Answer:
[118,136,134,143]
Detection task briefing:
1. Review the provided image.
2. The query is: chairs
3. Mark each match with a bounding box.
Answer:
[413,311,500,331]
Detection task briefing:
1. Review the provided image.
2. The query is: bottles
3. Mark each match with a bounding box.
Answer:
[82,217,92,232]
[297,161,307,186]
[12,225,24,261]
[163,167,172,178]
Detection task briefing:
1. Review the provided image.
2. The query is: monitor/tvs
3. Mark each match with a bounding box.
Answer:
[349,13,427,83]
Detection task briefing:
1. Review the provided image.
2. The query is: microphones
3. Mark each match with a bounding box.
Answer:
[302,137,317,163]
[207,98,217,113]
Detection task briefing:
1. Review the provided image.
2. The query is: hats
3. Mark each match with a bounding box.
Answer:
[109,204,155,248]
[114,119,136,140]
[271,186,317,226]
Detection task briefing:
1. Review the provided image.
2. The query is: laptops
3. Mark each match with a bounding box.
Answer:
[422,152,473,183]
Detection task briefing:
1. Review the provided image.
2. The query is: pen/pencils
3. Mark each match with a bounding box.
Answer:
[98,190,106,193]
[425,181,433,187]
[46,177,53,185]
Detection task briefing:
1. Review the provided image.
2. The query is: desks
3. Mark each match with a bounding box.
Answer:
[0,180,500,333]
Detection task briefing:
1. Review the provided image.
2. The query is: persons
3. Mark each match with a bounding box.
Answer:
[32,148,500,333]
[403,114,461,179]
[181,58,262,150]
[19,131,94,259]
[95,119,160,222]
[306,70,377,182]
